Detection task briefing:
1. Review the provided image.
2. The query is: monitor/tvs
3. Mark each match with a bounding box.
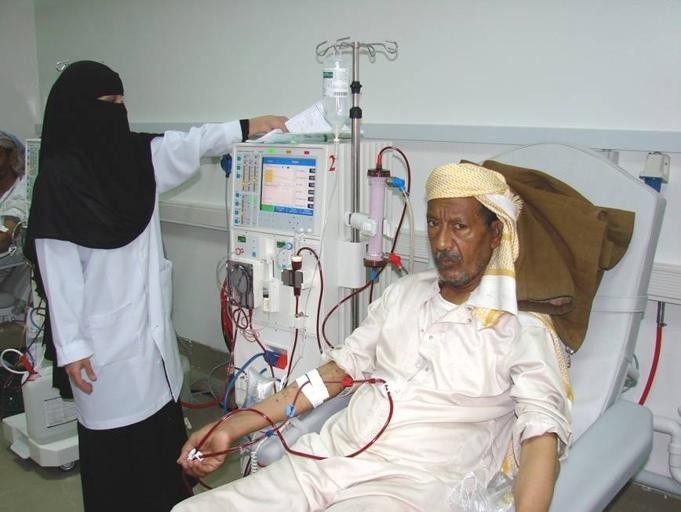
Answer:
[233,146,326,237]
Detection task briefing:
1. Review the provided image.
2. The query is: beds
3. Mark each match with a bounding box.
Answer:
[255,141,666,511]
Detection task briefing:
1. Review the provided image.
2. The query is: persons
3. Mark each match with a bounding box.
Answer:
[22,60,290,512]
[0,130,25,254]
[170,163,573,511]
